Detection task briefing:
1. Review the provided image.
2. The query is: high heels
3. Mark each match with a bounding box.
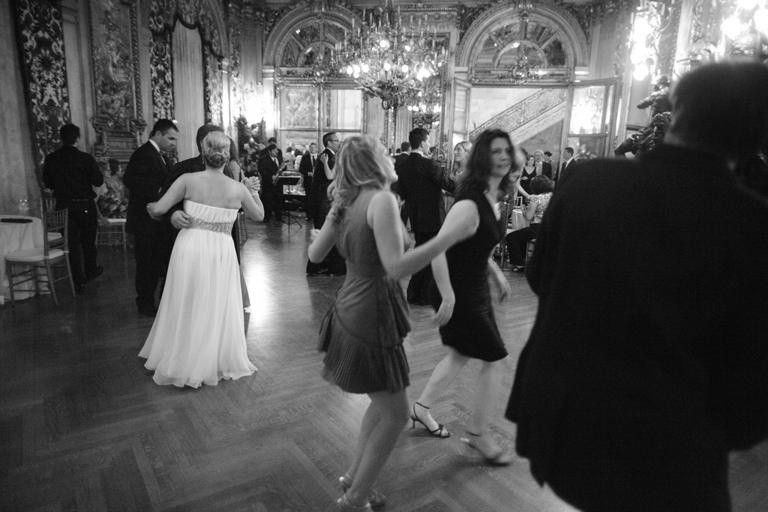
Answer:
[335,495,372,512]
[410,402,450,438]
[338,473,386,506]
[462,432,509,466]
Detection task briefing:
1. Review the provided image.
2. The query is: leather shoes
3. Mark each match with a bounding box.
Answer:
[87,266,102,276]
[512,267,523,272]
[139,308,157,317]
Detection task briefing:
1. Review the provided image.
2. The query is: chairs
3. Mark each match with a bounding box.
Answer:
[525,239,536,272]
[95,201,127,249]
[5,207,76,306]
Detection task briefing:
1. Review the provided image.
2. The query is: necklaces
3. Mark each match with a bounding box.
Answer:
[455,169,465,175]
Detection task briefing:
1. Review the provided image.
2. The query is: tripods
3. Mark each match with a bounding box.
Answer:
[274,185,302,236]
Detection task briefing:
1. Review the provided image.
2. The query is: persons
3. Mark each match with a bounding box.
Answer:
[123,119,180,315]
[311,131,341,275]
[409,129,513,466]
[92,158,129,216]
[319,153,336,181]
[498,147,575,273]
[307,137,479,511]
[160,124,241,265]
[138,131,265,389]
[445,141,471,215]
[42,124,103,294]
[258,138,317,222]
[388,142,410,228]
[504,62,767,512]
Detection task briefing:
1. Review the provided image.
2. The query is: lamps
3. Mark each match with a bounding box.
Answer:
[331,0,449,128]
